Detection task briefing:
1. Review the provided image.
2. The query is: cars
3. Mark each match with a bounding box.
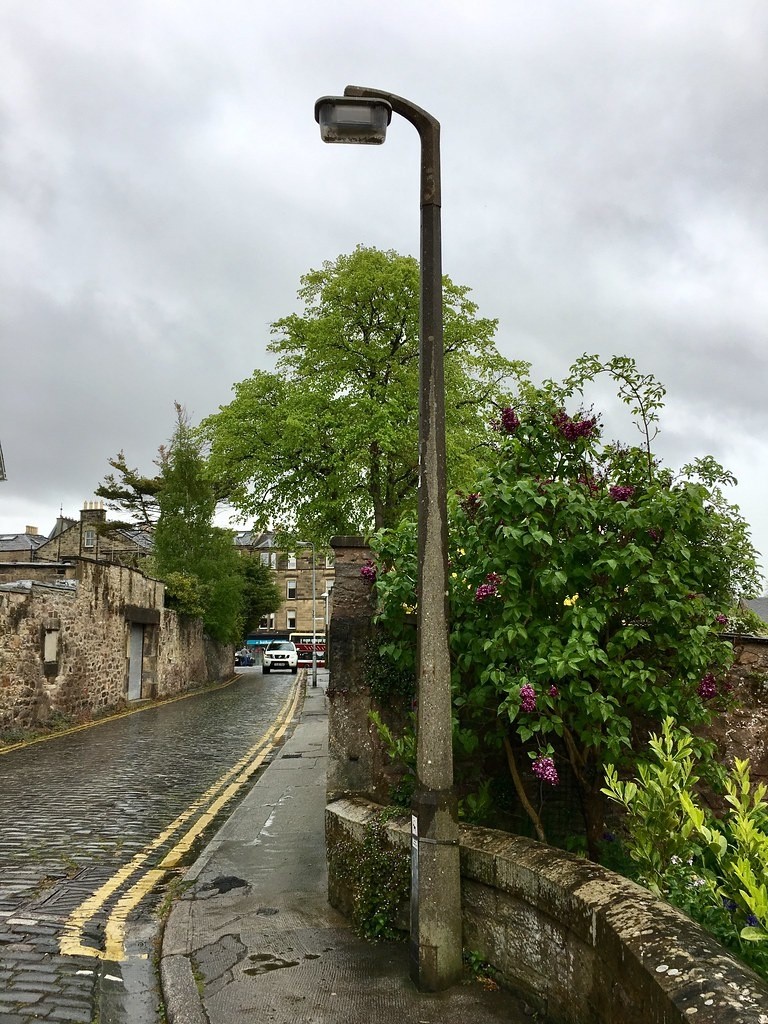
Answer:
[235,649,255,666]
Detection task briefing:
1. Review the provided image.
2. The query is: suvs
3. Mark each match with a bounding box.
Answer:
[261,639,300,674]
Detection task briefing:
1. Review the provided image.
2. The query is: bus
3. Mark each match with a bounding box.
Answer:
[289,633,325,668]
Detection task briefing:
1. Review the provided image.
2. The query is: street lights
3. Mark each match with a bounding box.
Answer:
[320,592,328,668]
[297,541,316,689]
[312,83,464,987]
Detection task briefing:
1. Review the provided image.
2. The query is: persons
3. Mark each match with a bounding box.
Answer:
[241,646,249,666]
